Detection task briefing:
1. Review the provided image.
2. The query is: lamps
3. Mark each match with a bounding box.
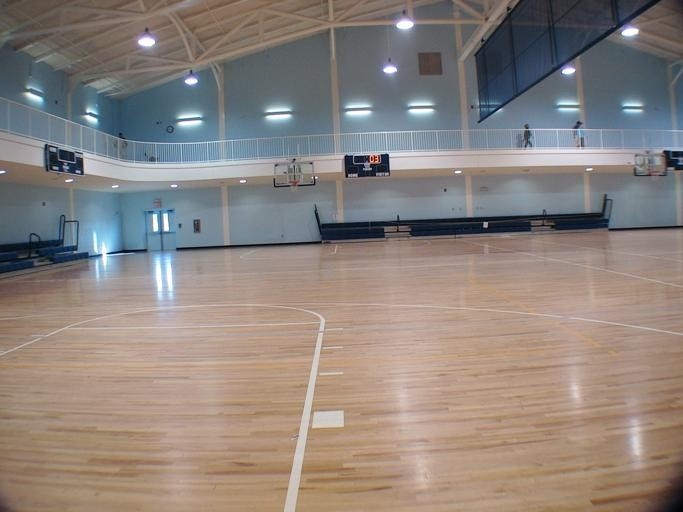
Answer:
[552,102,646,117]
[80,111,97,121]
[184,69,198,85]
[395,0,415,30]
[621,23,640,36]
[24,87,45,98]
[175,99,438,128]
[561,63,576,75]
[137,27,156,47]
[382,15,397,74]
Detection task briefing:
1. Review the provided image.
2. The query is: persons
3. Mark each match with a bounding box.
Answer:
[577,122,585,147]
[522,124,533,148]
[572,120,580,147]
[113,132,128,160]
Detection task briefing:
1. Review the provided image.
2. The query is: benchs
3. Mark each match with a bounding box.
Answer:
[314,192,612,243]
[1,215,89,274]
